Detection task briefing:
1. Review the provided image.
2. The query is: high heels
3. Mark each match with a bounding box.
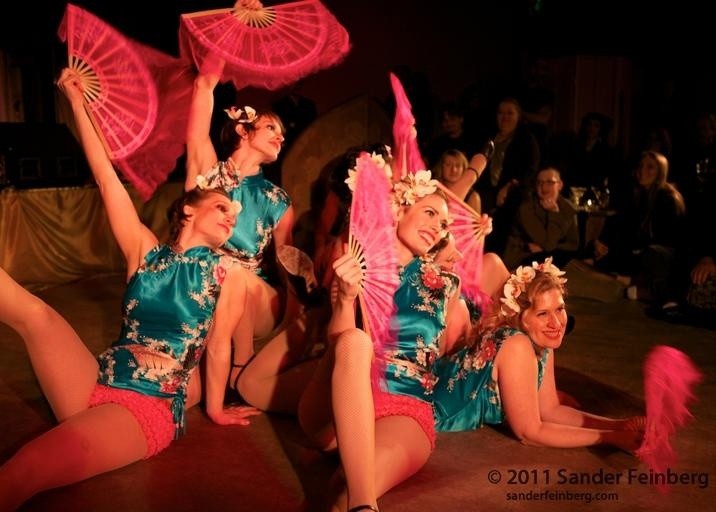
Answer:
[273,243,329,308]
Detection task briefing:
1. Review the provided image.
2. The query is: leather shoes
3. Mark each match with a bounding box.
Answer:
[478,139,497,164]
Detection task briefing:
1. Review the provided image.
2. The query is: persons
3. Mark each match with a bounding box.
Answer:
[0,64,263,512]
[233,92,716,512]
[179,0,321,395]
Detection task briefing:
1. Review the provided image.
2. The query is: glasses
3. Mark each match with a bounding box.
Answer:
[536,179,558,187]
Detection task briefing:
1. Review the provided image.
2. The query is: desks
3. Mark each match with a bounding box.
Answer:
[577,211,616,248]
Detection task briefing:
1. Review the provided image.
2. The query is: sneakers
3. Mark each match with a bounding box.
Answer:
[626,283,682,311]
